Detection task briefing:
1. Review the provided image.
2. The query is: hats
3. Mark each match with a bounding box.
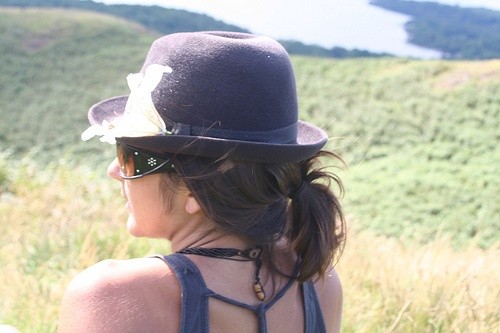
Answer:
[88,30,327,162]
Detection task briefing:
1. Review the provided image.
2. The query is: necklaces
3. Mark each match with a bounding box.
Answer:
[172,242,270,302]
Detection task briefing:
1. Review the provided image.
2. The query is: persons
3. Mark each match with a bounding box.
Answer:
[57,31,344,332]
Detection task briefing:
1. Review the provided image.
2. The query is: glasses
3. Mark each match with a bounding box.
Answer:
[115,142,177,180]
